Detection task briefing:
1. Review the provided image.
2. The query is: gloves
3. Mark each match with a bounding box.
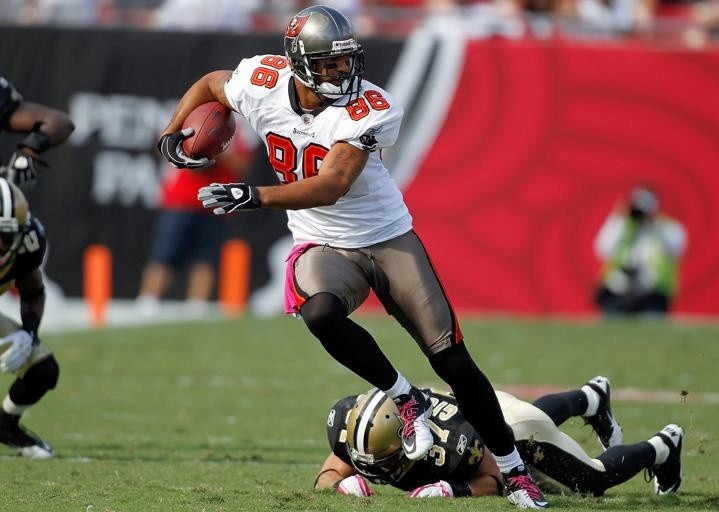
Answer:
[198,183,261,215]
[157,127,216,169]
[0,328,34,373]
[409,480,453,497]
[337,474,373,497]
[7,152,37,189]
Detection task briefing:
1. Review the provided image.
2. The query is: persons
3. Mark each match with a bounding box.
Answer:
[157,5,551,509]
[0,76,75,186]
[313,376,686,499]
[0,177,59,460]
[136,113,264,324]
[592,185,687,312]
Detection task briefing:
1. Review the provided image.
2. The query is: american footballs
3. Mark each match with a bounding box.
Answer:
[181,101,236,160]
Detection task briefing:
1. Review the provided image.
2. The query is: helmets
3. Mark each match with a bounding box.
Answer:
[0,177,31,278]
[345,388,414,484]
[284,5,366,107]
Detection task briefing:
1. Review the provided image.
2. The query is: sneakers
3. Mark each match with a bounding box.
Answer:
[0,425,53,458]
[585,376,623,451]
[644,423,683,494]
[393,384,434,461]
[502,465,548,509]
